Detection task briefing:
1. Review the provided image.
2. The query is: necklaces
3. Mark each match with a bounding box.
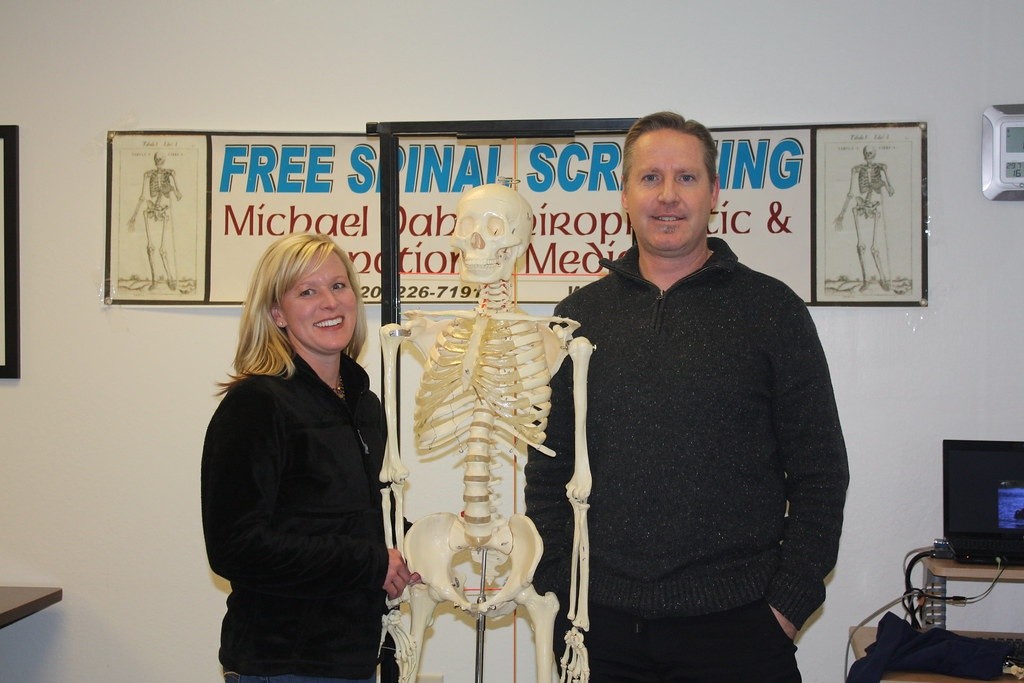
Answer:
[332,375,345,400]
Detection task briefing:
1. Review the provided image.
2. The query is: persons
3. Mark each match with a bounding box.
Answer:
[525,111,850,683]
[200,232,422,683]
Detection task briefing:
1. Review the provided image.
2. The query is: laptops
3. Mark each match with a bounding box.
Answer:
[942,439,1024,558]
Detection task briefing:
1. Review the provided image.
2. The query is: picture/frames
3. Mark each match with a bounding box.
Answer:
[0,125,21,379]
[105,120,929,306]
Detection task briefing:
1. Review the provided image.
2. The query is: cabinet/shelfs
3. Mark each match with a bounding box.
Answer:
[850,547,1024,683]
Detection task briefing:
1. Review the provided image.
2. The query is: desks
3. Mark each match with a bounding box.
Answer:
[0,586,63,628]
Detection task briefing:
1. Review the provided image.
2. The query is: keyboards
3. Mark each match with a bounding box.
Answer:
[979,637,1024,663]
